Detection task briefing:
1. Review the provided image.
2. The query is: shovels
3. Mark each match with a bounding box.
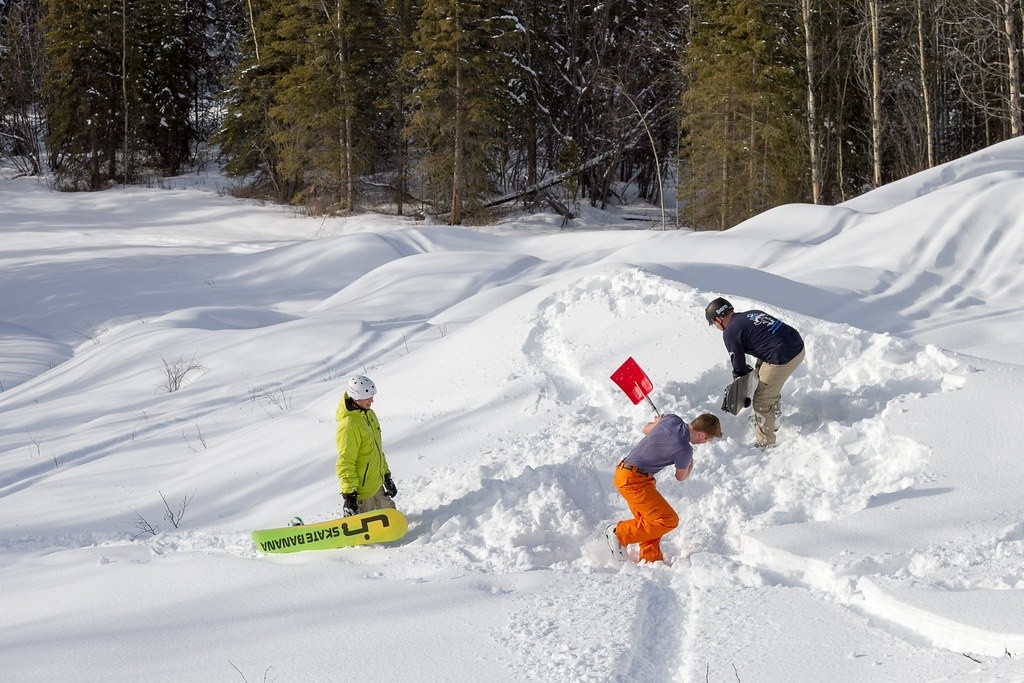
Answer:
[609,358,695,472]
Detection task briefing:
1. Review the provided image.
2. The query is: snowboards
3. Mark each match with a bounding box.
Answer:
[251,506,409,555]
[720,367,760,416]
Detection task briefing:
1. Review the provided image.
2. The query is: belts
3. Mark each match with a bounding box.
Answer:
[623,465,648,475]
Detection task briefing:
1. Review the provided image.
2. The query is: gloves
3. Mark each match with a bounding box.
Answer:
[743,397,751,408]
[382,473,397,498]
[342,490,358,517]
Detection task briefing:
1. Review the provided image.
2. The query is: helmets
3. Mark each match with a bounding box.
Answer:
[346,375,377,400]
[705,297,734,326]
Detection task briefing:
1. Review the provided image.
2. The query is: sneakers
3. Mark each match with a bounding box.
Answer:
[606,524,625,561]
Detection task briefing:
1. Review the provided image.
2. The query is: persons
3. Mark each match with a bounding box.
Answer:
[705,297,805,449]
[335,375,398,518]
[606,413,724,563]
